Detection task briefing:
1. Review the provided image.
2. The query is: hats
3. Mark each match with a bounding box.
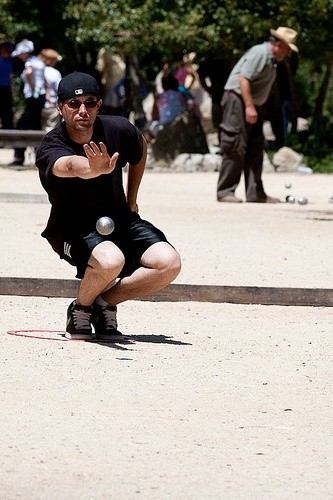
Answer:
[10,38,35,57]
[57,71,99,103]
[269,27,300,54]
[41,49,63,61]
[0,40,14,53]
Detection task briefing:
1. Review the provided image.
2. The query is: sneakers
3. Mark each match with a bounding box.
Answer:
[90,300,124,340]
[65,299,94,340]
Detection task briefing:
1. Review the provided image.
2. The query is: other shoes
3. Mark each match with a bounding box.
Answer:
[256,193,281,203]
[217,195,242,203]
[6,160,24,169]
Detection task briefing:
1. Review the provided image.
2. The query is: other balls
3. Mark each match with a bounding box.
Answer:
[286,195,295,203]
[284,182,291,189]
[96,216,115,236]
[297,196,307,205]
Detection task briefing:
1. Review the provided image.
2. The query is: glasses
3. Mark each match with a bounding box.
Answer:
[62,98,99,109]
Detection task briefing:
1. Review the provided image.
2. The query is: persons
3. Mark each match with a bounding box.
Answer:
[0,34,308,166]
[216,26,298,203]
[35,71,181,340]
